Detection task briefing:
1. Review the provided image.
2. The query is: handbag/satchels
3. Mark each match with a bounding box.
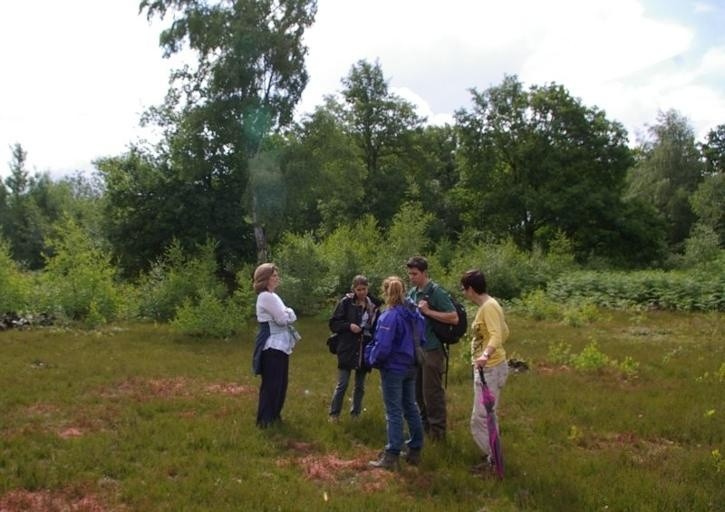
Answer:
[415,346,426,367]
[326,333,338,354]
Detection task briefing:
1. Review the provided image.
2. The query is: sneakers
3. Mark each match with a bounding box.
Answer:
[328,412,339,425]
[350,412,360,419]
[473,465,501,482]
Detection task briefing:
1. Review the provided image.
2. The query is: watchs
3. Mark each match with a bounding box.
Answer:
[482,350,492,358]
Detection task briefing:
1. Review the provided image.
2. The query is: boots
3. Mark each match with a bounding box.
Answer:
[403,447,422,466]
[369,453,401,473]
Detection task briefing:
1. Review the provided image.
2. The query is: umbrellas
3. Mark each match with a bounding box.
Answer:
[477,364,506,481]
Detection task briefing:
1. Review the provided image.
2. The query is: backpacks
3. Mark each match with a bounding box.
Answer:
[423,284,467,387]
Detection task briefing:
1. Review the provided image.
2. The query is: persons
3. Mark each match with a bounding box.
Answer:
[458,270,512,472]
[403,254,461,449]
[328,274,380,425]
[362,277,431,470]
[251,261,302,433]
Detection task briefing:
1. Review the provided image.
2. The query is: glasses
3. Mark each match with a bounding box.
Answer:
[460,286,470,292]
